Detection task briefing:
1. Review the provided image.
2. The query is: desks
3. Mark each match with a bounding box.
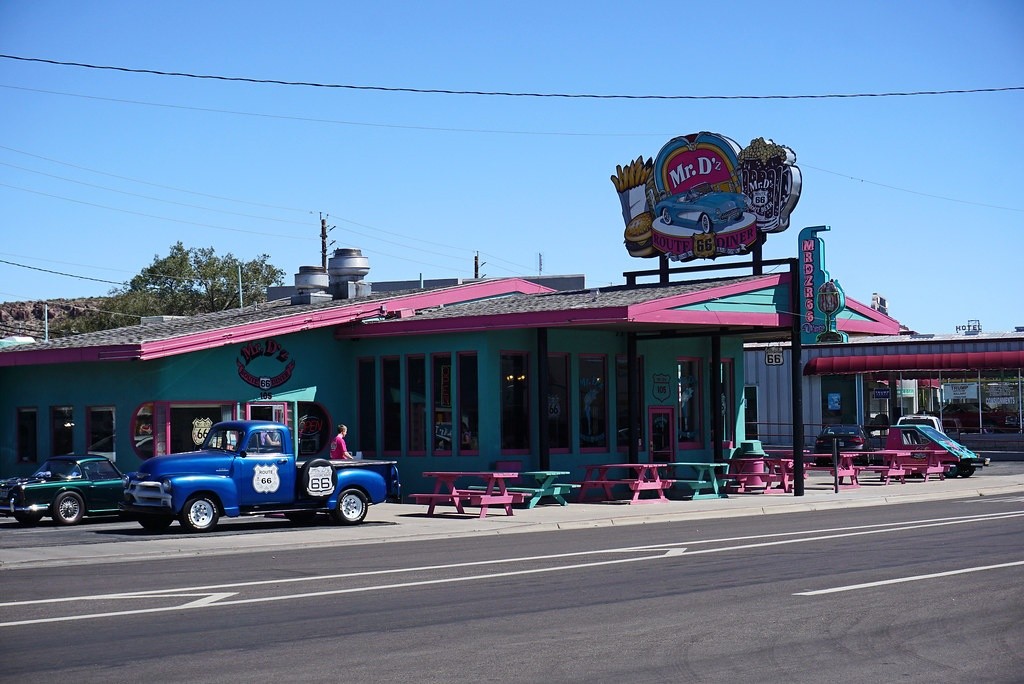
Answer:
[722,458,794,493]
[577,464,669,504]
[834,450,911,486]
[764,449,811,481]
[787,453,860,489]
[519,470,571,509]
[669,461,728,500]
[876,449,948,482]
[422,472,519,518]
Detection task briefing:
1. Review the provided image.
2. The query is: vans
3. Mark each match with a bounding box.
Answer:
[895,414,945,435]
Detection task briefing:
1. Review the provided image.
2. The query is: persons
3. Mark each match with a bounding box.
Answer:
[330,424,354,460]
[260,432,279,446]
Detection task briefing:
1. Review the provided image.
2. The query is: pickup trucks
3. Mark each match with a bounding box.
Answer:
[118,419,401,533]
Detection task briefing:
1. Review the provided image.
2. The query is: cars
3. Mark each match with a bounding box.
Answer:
[813,424,876,466]
[917,403,1020,434]
[0,455,131,525]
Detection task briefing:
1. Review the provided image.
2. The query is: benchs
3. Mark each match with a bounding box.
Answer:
[407,449,951,521]
